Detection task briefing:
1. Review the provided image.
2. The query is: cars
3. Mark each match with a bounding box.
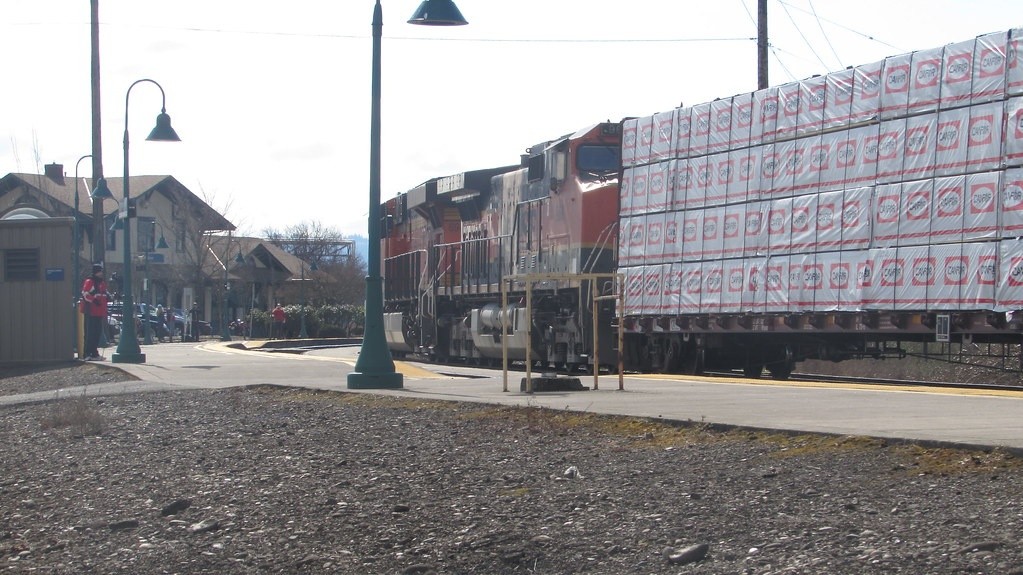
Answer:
[102,299,213,340]
[230,320,252,337]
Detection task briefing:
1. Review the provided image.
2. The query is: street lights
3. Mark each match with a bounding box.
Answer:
[347,0,473,391]
[71,153,112,351]
[142,221,169,346]
[219,238,247,343]
[110,78,183,365]
[299,253,319,338]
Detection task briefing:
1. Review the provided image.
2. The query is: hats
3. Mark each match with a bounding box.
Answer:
[93,264,102,273]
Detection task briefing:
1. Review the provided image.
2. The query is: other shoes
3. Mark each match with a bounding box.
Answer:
[91,354,106,361]
[85,356,91,361]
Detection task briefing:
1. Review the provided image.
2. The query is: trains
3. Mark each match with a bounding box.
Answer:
[382,27,1022,371]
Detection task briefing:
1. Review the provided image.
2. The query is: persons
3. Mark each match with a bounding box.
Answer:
[167,304,176,342]
[80,264,116,360]
[155,304,167,343]
[271,303,285,340]
[187,301,202,342]
